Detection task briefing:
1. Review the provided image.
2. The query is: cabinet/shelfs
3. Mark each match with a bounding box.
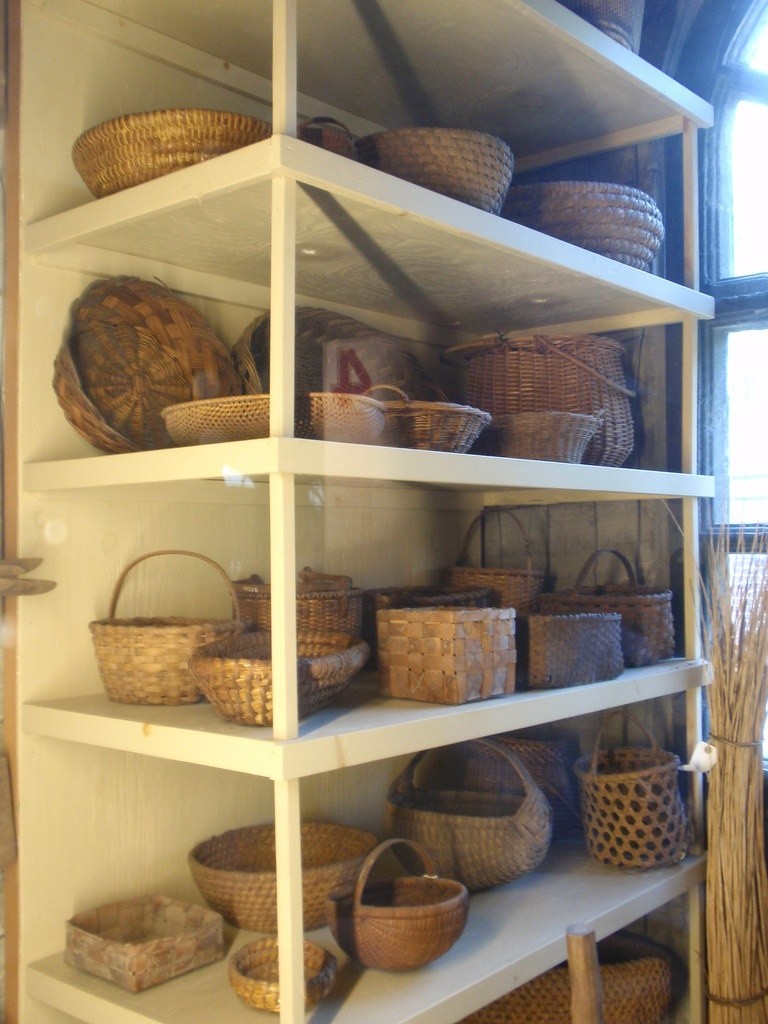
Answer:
[17,0,726,1024]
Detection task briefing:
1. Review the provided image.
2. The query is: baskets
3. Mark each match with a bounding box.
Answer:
[65,894,225,994]
[228,935,338,1012]
[385,710,693,895]
[188,822,385,935]
[230,508,676,705]
[186,626,371,727]
[50,275,638,466]
[87,549,254,707]
[560,0,646,54]
[323,838,470,973]
[458,933,673,1024]
[70,108,664,274]
